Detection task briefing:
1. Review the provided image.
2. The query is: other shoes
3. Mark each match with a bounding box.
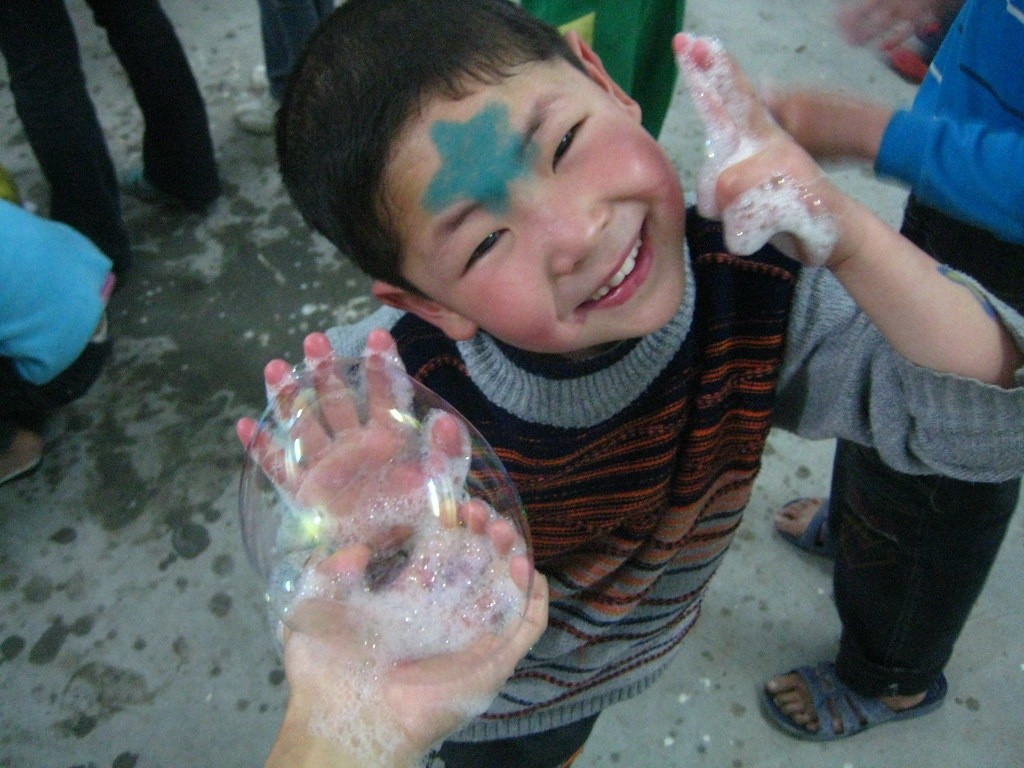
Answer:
[236,103,282,134]
[130,167,222,208]
[0,432,43,487]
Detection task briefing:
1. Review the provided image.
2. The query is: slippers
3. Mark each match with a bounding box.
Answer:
[774,497,841,560]
[759,660,948,741]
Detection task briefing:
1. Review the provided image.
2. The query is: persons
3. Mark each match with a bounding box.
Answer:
[518,1,686,144]
[259,497,549,766]
[2,0,222,296]
[0,197,115,484]
[759,0,1023,743]
[235,1,339,137]
[235,0,1022,768]
